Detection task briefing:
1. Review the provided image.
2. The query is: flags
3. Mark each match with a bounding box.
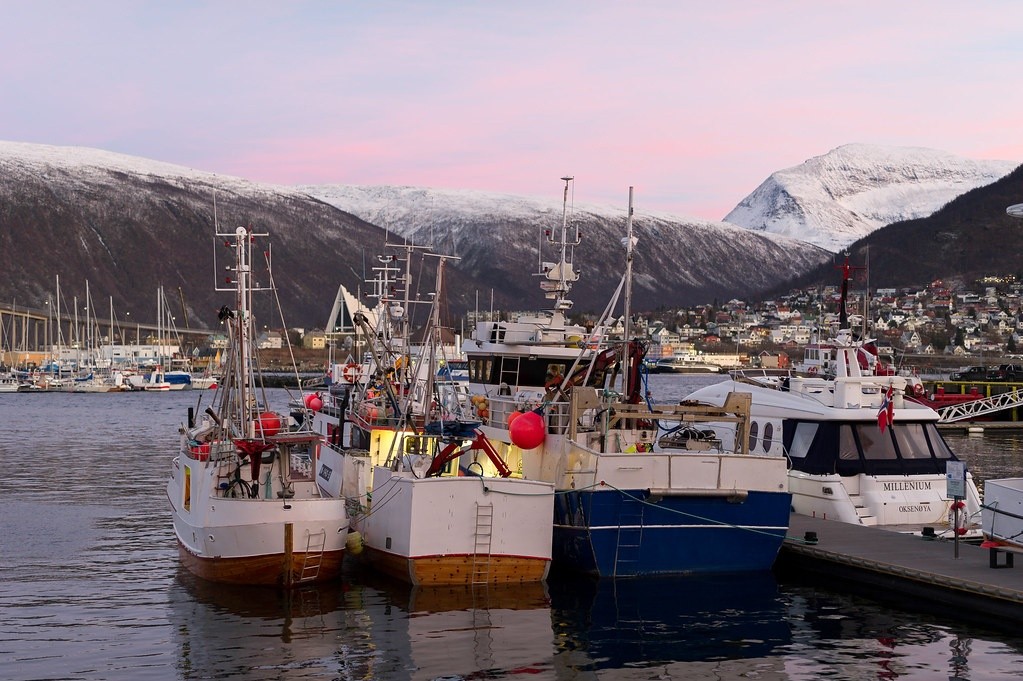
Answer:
[878,385,895,434]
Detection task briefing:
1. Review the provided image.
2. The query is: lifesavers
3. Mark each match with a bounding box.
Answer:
[808,367,812,374]
[948,502,970,535]
[343,362,363,383]
[812,366,818,375]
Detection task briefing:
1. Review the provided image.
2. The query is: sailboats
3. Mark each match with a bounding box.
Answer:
[0,177,1022,594]
